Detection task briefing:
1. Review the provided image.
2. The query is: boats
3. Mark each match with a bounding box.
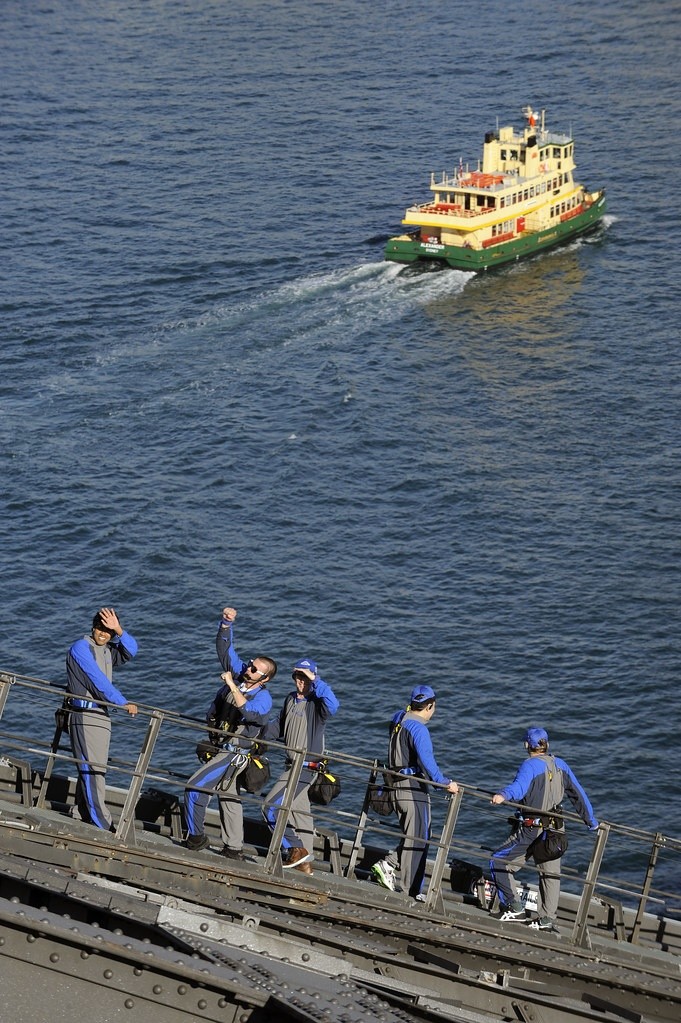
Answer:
[378,103,605,273]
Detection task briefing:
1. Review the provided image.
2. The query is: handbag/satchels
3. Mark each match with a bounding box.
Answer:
[309,769,341,805]
[240,757,271,795]
[531,827,568,864]
[197,740,219,762]
[369,783,394,816]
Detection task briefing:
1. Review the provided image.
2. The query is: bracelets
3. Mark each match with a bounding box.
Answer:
[231,688,240,693]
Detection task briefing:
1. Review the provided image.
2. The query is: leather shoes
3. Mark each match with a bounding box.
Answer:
[282,846,315,876]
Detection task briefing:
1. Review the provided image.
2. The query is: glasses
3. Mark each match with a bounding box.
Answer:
[248,659,265,677]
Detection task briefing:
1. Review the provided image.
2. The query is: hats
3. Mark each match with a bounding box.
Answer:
[411,685,440,704]
[520,726,549,749]
[292,657,317,680]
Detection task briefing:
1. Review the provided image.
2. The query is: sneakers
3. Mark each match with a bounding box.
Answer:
[525,916,552,933]
[371,859,396,892]
[415,893,426,902]
[179,833,211,851]
[489,909,527,922]
[217,848,246,863]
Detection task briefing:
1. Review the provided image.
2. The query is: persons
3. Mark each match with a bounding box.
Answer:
[66,608,138,834]
[180,607,278,862]
[489,726,599,932]
[260,657,340,876]
[370,685,458,903]
